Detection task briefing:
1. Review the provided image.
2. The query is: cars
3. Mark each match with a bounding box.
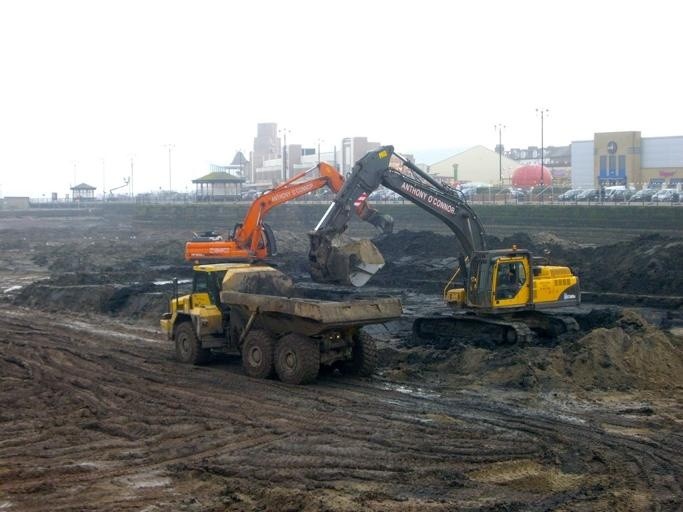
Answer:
[241,189,329,200]
[558,185,683,203]
[368,181,525,199]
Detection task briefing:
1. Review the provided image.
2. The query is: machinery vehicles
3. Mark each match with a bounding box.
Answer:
[308,145,582,346]
[185,162,394,262]
[158,260,403,385]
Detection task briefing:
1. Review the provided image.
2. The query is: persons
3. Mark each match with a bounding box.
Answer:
[601,188,606,202]
[497,268,516,298]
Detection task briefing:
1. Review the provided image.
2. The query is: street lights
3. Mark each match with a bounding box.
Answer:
[535,108,549,200]
[278,127,292,181]
[494,122,506,186]
[163,143,175,196]
